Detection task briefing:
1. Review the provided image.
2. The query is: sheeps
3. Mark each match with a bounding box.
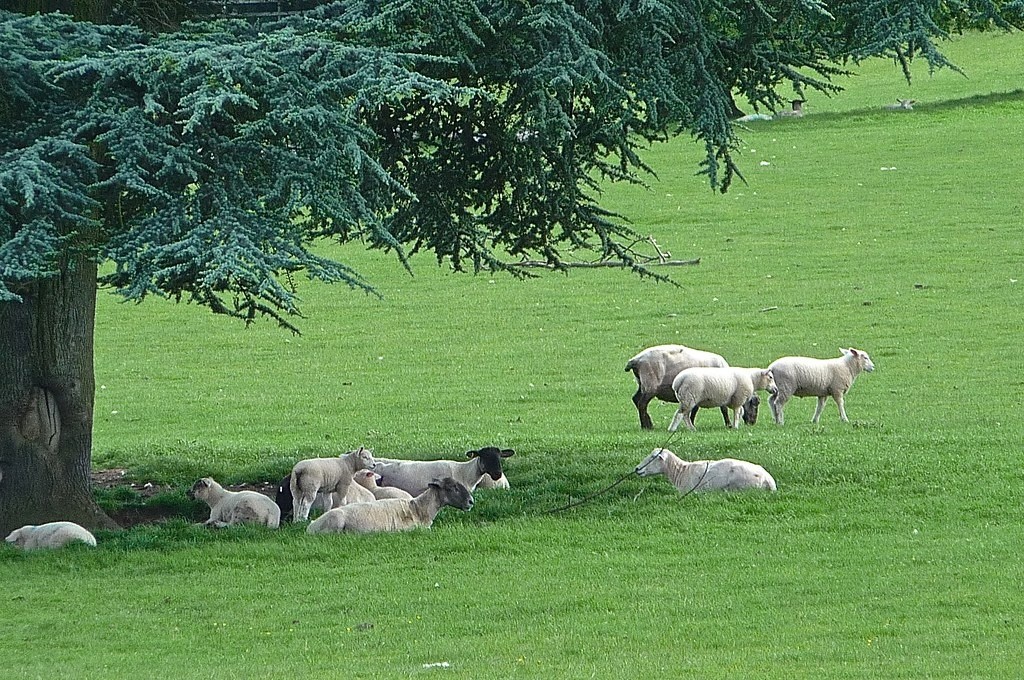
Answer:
[634,448,777,494]
[5,522,98,550]
[186,476,281,528]
[624,344,875,432]
[290,446,514,533]
